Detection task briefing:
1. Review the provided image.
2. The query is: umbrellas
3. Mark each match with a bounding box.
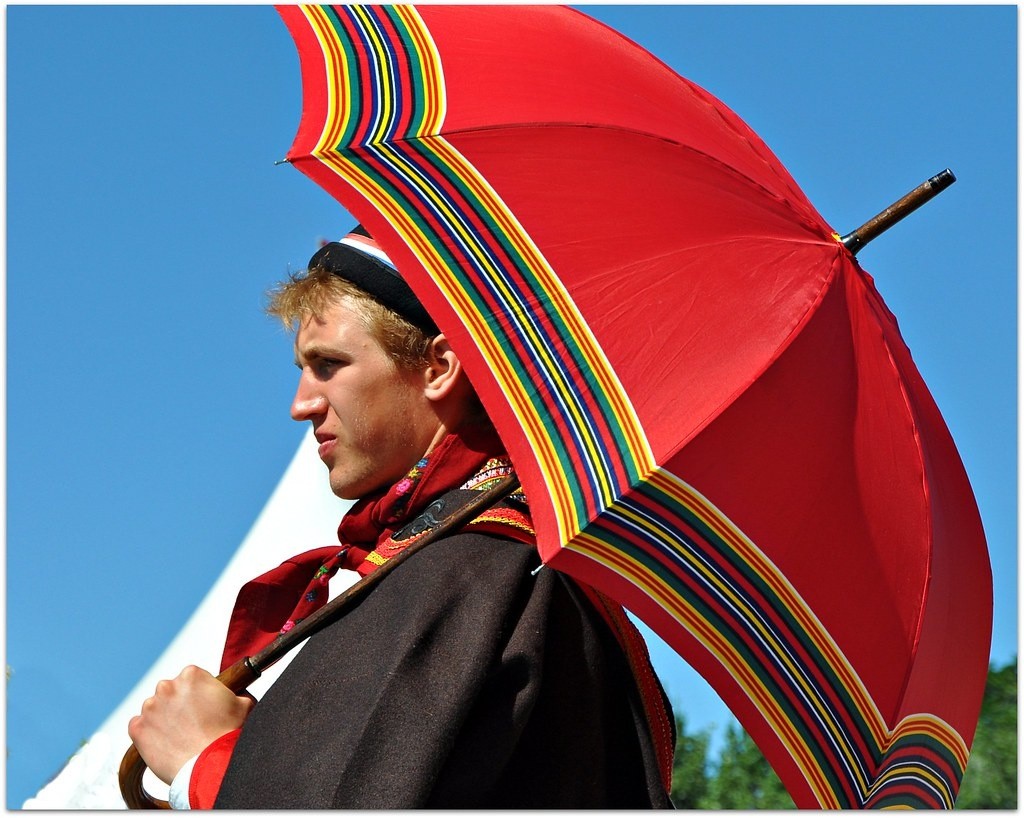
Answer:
[275,4,997,810]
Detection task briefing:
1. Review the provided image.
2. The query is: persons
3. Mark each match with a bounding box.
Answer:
[127,223,677,811]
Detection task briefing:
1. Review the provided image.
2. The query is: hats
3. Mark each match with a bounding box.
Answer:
[309,223,441,338]
